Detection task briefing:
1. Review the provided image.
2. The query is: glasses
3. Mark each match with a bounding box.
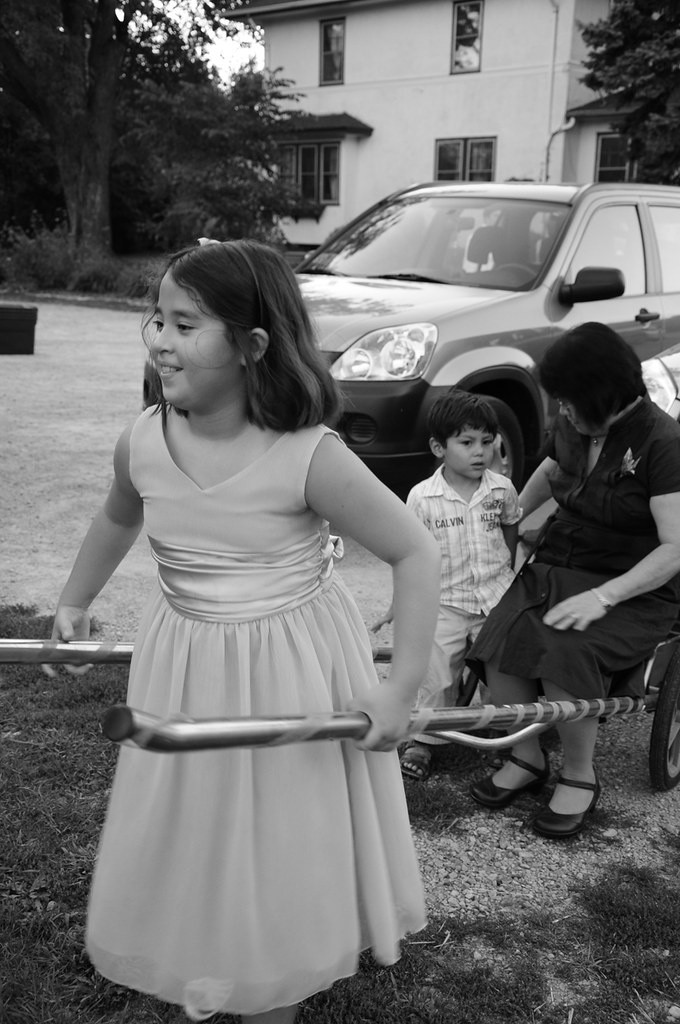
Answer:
[556,397,575,406]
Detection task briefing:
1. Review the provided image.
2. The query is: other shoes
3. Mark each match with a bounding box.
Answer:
[184,977,232,1022]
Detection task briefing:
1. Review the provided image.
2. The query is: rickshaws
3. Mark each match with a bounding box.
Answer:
[1,506,680,794]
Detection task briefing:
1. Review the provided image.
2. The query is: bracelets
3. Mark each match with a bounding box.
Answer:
[590,588,613,613]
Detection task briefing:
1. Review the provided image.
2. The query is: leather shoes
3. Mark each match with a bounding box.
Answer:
[531,767,600,838]
[469,747,550,807]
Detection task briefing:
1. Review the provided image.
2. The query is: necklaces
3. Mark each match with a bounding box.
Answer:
[592,432,606,447]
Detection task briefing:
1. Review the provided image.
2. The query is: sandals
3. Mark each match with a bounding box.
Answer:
[399,745,434,779]
[487,736,510,767]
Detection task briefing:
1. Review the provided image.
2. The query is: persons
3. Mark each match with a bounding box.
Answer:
[464,322,680,836]
[370,389,523,778]
[462,205,503,274]
[42,238,440,1024]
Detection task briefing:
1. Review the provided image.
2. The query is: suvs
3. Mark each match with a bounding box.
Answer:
[139,181,680,505]
